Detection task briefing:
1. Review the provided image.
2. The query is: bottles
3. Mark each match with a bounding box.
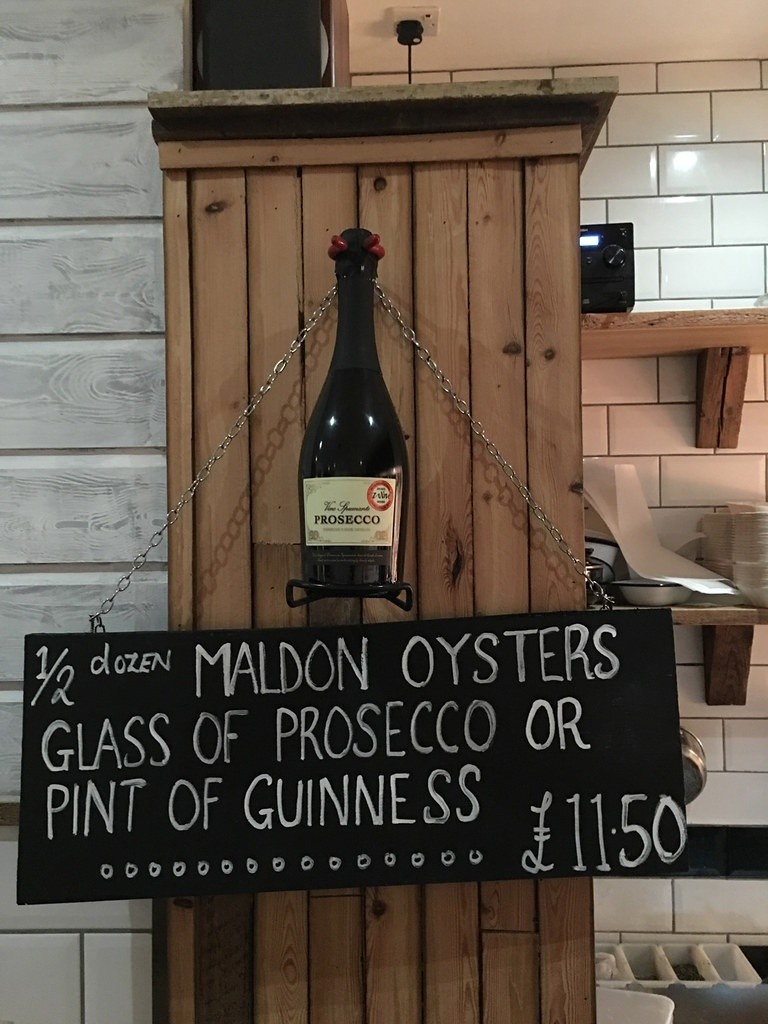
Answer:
[299,228,411,599]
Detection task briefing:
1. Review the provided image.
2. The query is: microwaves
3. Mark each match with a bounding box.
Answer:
[578,221,635,313]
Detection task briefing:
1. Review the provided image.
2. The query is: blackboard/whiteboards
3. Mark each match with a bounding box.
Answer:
[14,605,689,907]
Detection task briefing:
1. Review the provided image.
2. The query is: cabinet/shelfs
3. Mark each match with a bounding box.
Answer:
[581,307,768,705]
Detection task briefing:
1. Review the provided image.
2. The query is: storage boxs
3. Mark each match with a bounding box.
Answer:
[594,941,763,1024]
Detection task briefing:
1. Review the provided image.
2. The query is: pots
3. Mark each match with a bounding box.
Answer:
[680,727,708,804]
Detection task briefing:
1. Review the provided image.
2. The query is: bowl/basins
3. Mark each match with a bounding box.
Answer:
[698,502,768,609]
[610,579,692,606]
[585,528,619,567]
